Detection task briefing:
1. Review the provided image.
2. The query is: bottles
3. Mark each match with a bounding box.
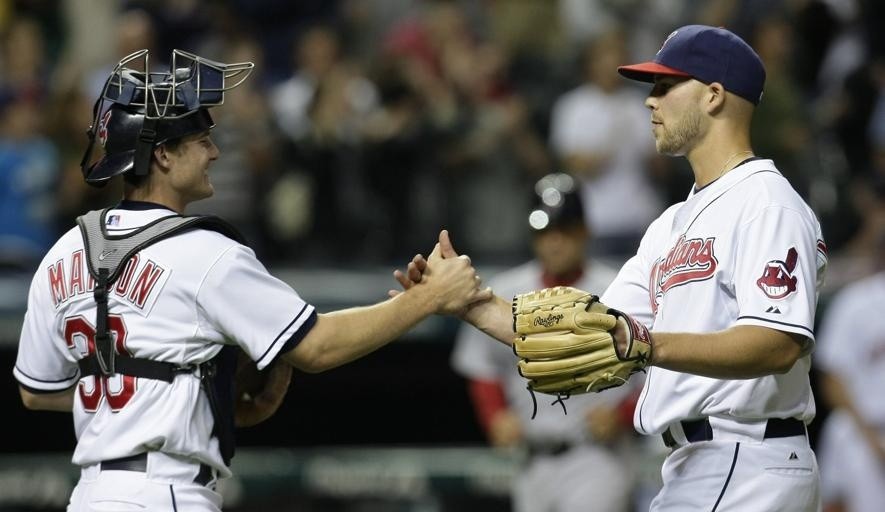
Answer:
[616,23,766,107]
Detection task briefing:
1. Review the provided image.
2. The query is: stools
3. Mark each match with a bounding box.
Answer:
[661,419,807,447]
[101,453,219,492]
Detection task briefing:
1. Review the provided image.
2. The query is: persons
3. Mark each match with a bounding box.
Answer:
[13,49,492,511]
[388,25,827,509]
[0,0,882,510]
[811,269,883,505]
[448,173,645,509]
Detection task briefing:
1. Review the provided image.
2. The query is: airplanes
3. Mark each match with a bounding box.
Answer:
[511,286,651,395]
[232,346,294,429]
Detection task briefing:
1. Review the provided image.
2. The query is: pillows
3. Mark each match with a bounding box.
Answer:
[86,48,255,182]
